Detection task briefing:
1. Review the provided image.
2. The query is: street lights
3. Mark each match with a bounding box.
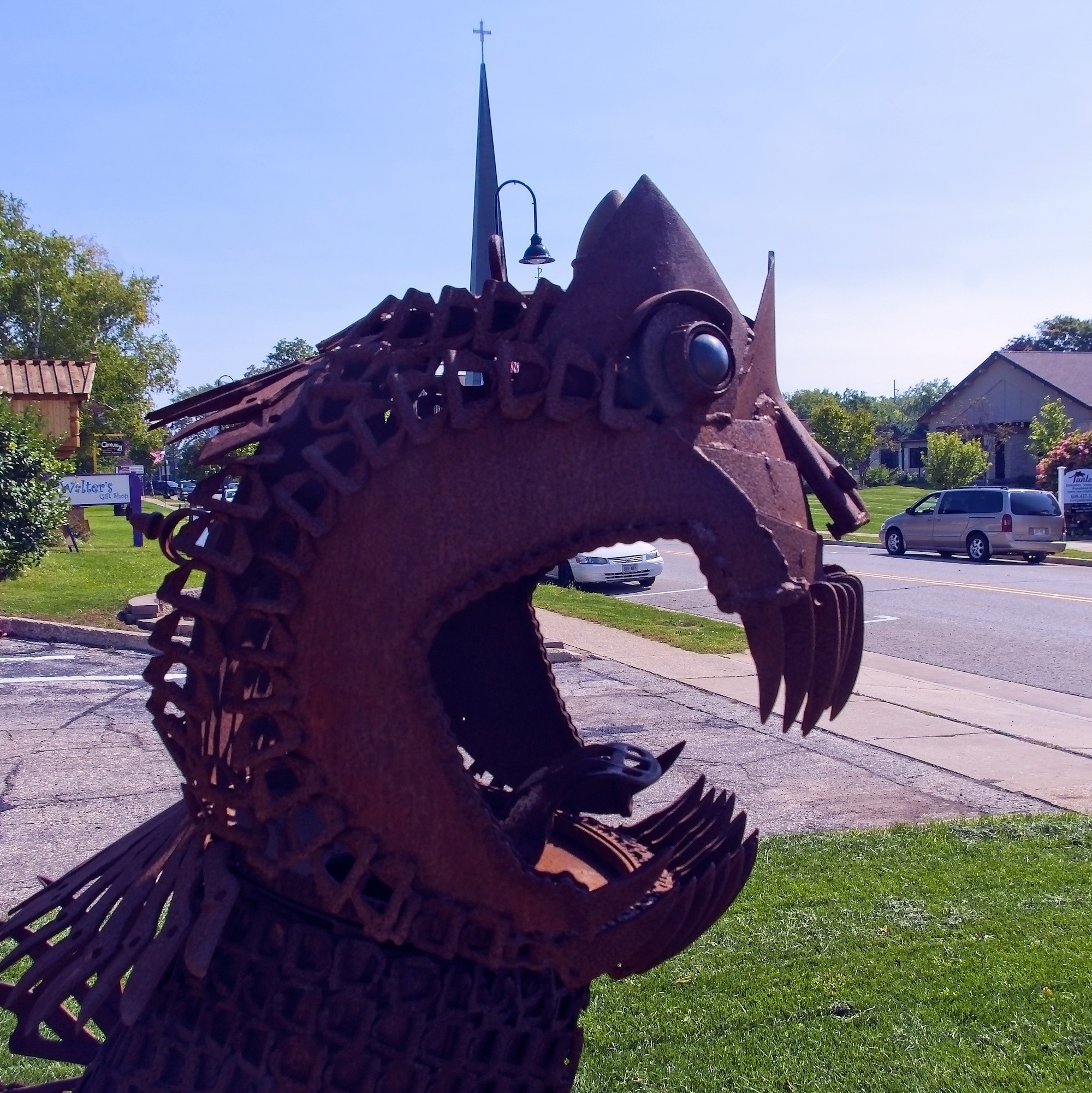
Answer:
[163,423,177,499]
[218,374,234,501]
[495,179,557,264]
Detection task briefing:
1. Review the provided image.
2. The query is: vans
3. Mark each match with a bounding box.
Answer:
[879,487,1068,563]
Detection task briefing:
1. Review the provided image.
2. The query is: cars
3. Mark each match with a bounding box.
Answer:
[542,540,665,589]
[178,480,199,502]
[147,479,179,497]
[212,482,240,502]
